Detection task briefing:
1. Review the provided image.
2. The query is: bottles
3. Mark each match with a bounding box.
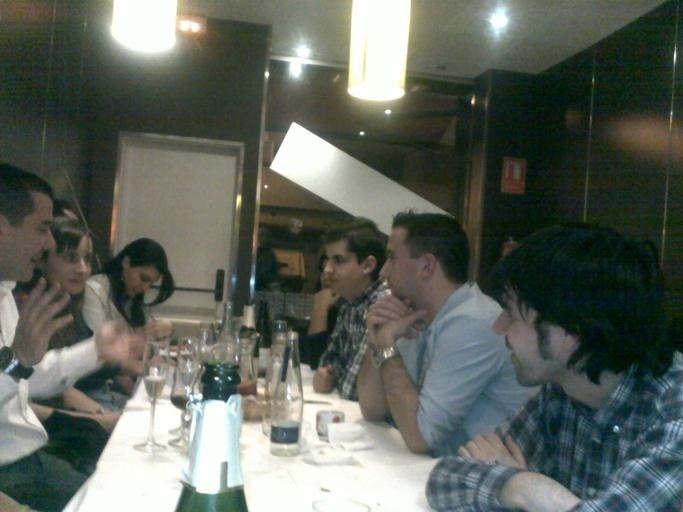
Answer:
[264,319,305,460]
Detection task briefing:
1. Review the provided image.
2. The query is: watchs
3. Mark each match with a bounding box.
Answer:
[372,345,400,369]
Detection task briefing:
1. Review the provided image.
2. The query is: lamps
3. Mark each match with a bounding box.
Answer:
[347,0,411,100]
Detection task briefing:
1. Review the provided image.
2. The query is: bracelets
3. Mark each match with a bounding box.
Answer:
[0,346,34,381]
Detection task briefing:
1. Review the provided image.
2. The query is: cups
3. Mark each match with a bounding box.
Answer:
[133,339,172,453]
[310,445,356,465]
[327,422,363,451]
[316,410,346,442]
[170,298,262,512]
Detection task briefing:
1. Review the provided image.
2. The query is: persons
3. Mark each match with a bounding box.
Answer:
[0,160,159,512]
[295,249,343,369]
[13,197,176,474]
[426,222,683,512]
[357,207,542,461]
[312,218,392,402]
